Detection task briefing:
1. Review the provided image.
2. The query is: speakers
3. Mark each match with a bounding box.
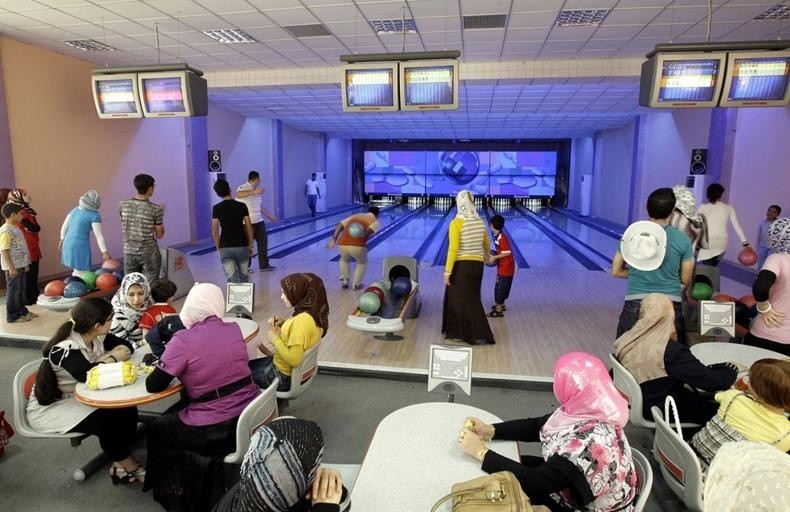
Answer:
[208,150,222,172]
[690,149,708,174]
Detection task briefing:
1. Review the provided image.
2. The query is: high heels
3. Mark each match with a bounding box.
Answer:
[107,464,137,485]
[129,463,148,487]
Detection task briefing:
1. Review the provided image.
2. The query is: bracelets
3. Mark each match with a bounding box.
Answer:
[487,425,495,438]
[756,301,773,315]
[110,355,119,363]
[478,450,488,463]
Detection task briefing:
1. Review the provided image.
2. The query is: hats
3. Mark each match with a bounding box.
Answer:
[619,220,668,272]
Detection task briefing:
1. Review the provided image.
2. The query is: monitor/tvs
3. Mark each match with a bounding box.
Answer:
[399,59,459,112]
[719,50,790,109]
[341,62,399,112]
[138,70,208,118]
[91,72,144,119]
[639,51,727,109]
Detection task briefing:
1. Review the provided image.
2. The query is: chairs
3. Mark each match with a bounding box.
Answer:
[650,405,705,512]
[629,446,654,512]
[270,415,361,498]
[261,339,324,416]
[13,358,88,448]
[225,375,280,466]
[608,351,703,453]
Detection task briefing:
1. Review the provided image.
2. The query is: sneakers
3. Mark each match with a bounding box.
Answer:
[352,284,366,290]
[259,265,277,272]
[12,315,31,323]
[341,282,350,289]
[246,267,256,273]
[26,312,39,318]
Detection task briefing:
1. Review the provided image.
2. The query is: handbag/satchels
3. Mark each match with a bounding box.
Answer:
[451,469,535,512]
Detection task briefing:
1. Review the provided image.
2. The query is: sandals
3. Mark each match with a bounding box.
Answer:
[491,304,507,311]
[486,310,506,318]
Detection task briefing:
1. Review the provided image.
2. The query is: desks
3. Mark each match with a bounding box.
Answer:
[689,341,790,418]
[346,401,523,512]
[72,317,261,483]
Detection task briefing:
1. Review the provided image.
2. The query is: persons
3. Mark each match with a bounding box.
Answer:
[110,273,177,348]
[212,179,254,320]
[442,190,495,345]
[143,283,262,512]
[697,440,790,511]
[7,188,40,318]
[57,190,111,275]
[757,205,782,272]
[213,415,350,512]
[688,358,790,467]
[608,292,746,427]
[247,273,329,392]
[0,203,38,323]
[327,205,381,291]
[694,184,749,266]
[235,171,278,273]
[743,218,790,359]
[485,214,515,317]
[26,298,146,484]
[612,187,695,338]
[120,174,167,284]
[458,352,638,512]
[303,172,321,217]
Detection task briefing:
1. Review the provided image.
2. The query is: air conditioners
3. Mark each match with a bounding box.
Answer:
[684,174,705,210]
[315,171,328,213]
[208,171,230,238]
[578,174,592,217]
